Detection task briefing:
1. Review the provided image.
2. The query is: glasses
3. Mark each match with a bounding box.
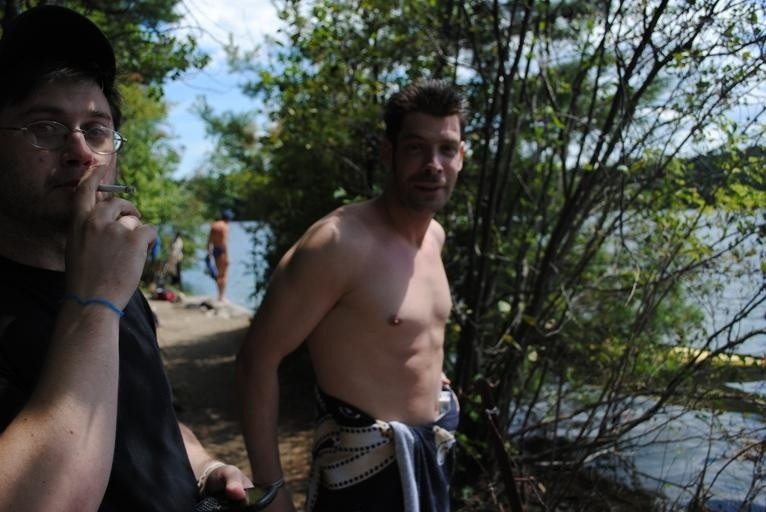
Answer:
[0,120,128,156]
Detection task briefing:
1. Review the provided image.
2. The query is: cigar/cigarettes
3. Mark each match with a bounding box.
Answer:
[71,181,134,193]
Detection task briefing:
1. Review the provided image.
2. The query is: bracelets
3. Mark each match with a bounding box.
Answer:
[195,460,224,495]
[61,294,125,318]
[252,479,284,492]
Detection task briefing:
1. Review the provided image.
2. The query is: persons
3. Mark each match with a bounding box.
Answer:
[232,77,466,512]
[0,3,255,512]
[144,226,160,282]
[167,230,183,290]
[205,213,228,301]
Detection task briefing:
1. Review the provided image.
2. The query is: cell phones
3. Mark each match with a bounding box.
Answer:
[193,483,278,512]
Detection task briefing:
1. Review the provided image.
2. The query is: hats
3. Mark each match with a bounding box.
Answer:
[1,5,116,104]
